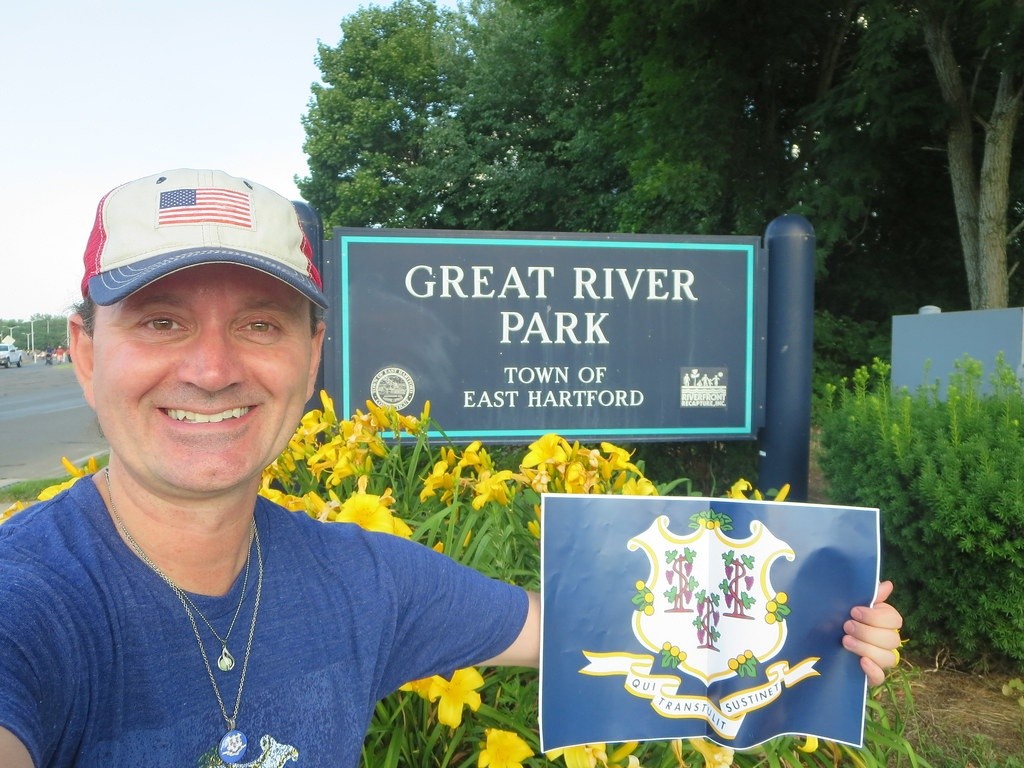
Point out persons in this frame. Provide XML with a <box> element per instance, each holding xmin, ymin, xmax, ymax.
<box><xmin>0</xmin><ymin>166</ymin><xmax>902</xmax><ymax>768</ymax></box>
<box><xmin>26</xmin><ymin>344</ymin><xmax>72</xmax><ymax>366</ymax></box>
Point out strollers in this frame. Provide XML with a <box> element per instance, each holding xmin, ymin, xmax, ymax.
<box><xmin>46</xmin><ymin>354</ymin><xmax>53</xmax><ymax>365</ymax></box>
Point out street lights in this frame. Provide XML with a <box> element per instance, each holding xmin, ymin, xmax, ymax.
<box><xmin>21</xmin><ymin>332</ymin><xmax>35</xmax><ymax>349</ymax></box>
<box><xmin>2</xmin><ymin>325</ymin><xmax>20</xmax><ymax>338</ymax></box>
<box><xmin>22</xmin><ymin>319</ymin><xmax>43</xmax><ymax>352</ymax></box>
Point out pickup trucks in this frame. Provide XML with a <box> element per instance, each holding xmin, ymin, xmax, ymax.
<box><xmin>0</xmin><ymin>344</ymin><xmax>22</xmax><ymax>368</ymax></box>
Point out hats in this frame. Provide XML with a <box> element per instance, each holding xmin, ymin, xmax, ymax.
<box><xmin>80</xmin><ymin>167</ymin><xmax>329</xmax><ymax>309</ymax></box>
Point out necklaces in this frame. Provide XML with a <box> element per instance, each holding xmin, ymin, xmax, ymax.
<box><xmin>103</xmin><ymin>461</ymin><xmax>263</xmax><ymax>762</ymax></box>
